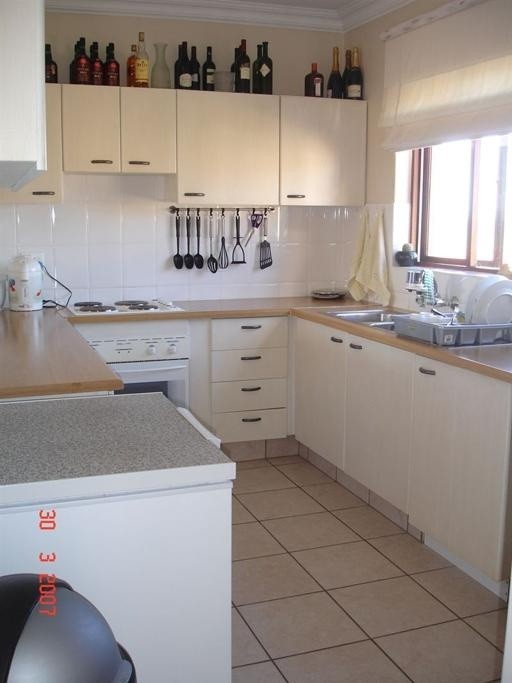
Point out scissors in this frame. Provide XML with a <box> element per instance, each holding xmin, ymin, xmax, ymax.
<box><xmin>245</xmin><ymin>214</ymin><xmax>263</xmax><ymax>248</ymax></box>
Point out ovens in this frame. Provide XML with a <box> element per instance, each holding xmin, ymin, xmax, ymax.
<box><xmin>105</xmin><ymin>359</ymin><xmax>188</xmax><ymax>407</ymax></box>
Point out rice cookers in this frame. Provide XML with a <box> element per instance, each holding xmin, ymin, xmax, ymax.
<box><xmin>6</xmin><ymin>255</ymin><xmax>46</xmax><ymax>311</ymax></box>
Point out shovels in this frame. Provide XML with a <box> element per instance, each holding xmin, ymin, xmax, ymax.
<box><xmin>260</xmin><ymin>211</ymin><xmax>272</xmax><ymax>269</ymax></box>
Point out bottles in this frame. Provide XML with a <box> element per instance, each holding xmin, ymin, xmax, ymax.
<box><xmin>305</xmin><ymin>62</ymin><xmax>324</xmax><ymax>96</ymax></box>
<box><xmin>327</xmin><ymin>46</ymin><xmax>363</xmax><ymax>98</ymax></box>
<box><xmin>70</xmin><ymin>30</ymin><xmax>273</xmax><ymax>94</ymax></box>
<box><xmin>46</xmin><ymin>43</ymin><xmax>58</xmax><ymax>82</ymax></box>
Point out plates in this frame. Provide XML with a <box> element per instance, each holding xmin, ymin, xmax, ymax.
<box><xmin>310</xmin><ymin>288</ymin><xmax>344</xmax><ymax>300</ymax></box>
<box><xmin>465</xmin><ymin>274</ymin><xmax>511</xmax><ymax>324</ymax></box>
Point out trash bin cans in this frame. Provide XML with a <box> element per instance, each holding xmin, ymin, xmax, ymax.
<box><xmin>0</xmin><ymin>575</ymin><xmax>137</xmax><ymax>683</ymax></box>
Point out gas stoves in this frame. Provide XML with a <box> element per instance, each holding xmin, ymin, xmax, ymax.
<box><xmin>66</xmin><ymin>297</ymin><xmax>191</xmax><ymax>360</ymax></box>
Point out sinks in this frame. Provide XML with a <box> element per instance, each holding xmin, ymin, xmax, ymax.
<box><xmin>370</xmin><ymin>324</ymin><xmax>395</xmax><ymax>332</ymax></box>
<box><xmin>323</xmin><ymin>309</ymin><xmax>405</xmax><ymax>323</ymax></box>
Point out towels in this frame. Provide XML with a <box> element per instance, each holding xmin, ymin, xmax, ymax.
<box><xmin>355</xmin><ymin>208</ymin><xmax>394</xmax><ymax>309</ymax></box>
<box><xmin>345</xmin><ymin>210</ymin><xmax>372</xmax><ymax>303</ymax></box>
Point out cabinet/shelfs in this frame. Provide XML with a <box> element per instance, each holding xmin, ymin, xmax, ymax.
<box><xmin>210</xmin><ymin>316</ymin><xmax>289</xmax><ymax>444</ymax></box>
<box><xmin>280</xmin><ymin>94</ymin><xmax>369</xmax><ymax>207</ymax></box>
<box><xmin>295</xmin><ymin>315</ymin><xmax>414</xmax><ymax>514</ymax></box>
<box><xmin>62</xmin><ymin>82</ymin><xmax>178</xmax><ymax>176</ymax></box>
<box><xmin>1</xmin><ymin>82</ymin><xmax>63</xmax><ymax>206</ymax></box>
<box><xmin>1</xmin><ymin>0</ymin><xmax>49</xmax><ymax>194</ymax></box>
<box><xmin>406</xmin><ymin>352</ymin><xmax>510</xmax><ymax>581</ymax></box>
<box><xmin>155</xmin><ymin>88</ymin><xmax>280</xmax><ymax>207</ymax></box>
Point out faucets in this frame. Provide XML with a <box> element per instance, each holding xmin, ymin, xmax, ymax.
<box><xmin>418</xmin><ymin>271</ymin><xmax>440</xmax><ymax>307</ymax></box>
